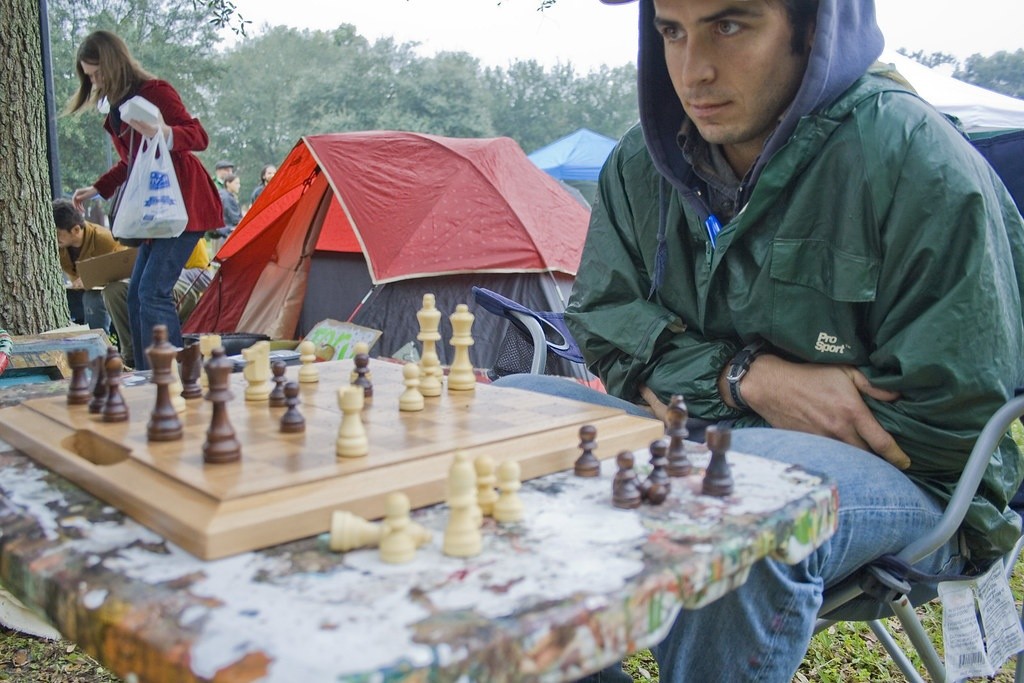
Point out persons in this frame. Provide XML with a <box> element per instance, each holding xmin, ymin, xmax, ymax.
<box><xmin>202</xmin><ymin>162</ymin><xmax>234</xmax><ymax>260</ymax></box>
<box><xmin>58</xmin><ymin>30</ymin><xmax>227</xmax><ymax>370</ymax></box>
<box><xmin>216</xmin><ymin>175</ymin><xmax>243</xmax><ymax>253</ymax></box>
<box><xmin>486</xmin><ymin>0</ymin><xmax>1024</xmax><ymax>683</ymax></box>
<box><xmin>178</xmin><ymin>238</ymin><xmax>214</xmax><ymax>294</ymax></box>
<box><xmin>52</xmin><ymin>198</ymin><xmax>129</xmax><ymax>335</ymax></box>
<box><xmin>250</xmin><ymin>164</ymin><xmax>277</xmax><ymax>206</ymax></box>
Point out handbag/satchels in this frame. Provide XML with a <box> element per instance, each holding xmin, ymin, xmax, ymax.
<box><xmin>111</xmin><ymin>127</ymin><xmax>189</xmax><ymax>239</ymax></box>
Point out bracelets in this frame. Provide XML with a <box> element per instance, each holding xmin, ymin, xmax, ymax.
<box><xmin>725</xmin><ymin>338</ymin><xmax>787</xmax><ymax>415</ymax></box>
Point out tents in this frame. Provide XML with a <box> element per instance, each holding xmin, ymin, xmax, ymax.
<box><xmin>175</xmin><ymin>45</ymin><xmax>1024</xmax><ymax>395</ymax></box>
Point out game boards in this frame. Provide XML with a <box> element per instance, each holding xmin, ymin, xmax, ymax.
<box><xmin>1</xmin><ymin>352</ymin><xmax>669</xmax><ymax>567</ymax></box>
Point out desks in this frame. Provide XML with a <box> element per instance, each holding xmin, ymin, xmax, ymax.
<box><xmin>0</xmin><ymin>355</ymin><xmax>838</xmax><ymax>683</ymax></box>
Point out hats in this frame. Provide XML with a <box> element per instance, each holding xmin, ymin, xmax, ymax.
<box><xmin>216</xmin><ymin>161</ymin><xmax>235</xmax><ymax>169</ymax></box>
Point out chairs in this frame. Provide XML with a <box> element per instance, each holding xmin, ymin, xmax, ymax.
<box><xmin>471</xmin><ymin>124</ymin><xmax>1024</xmax><ymax>683</ymax></box>
